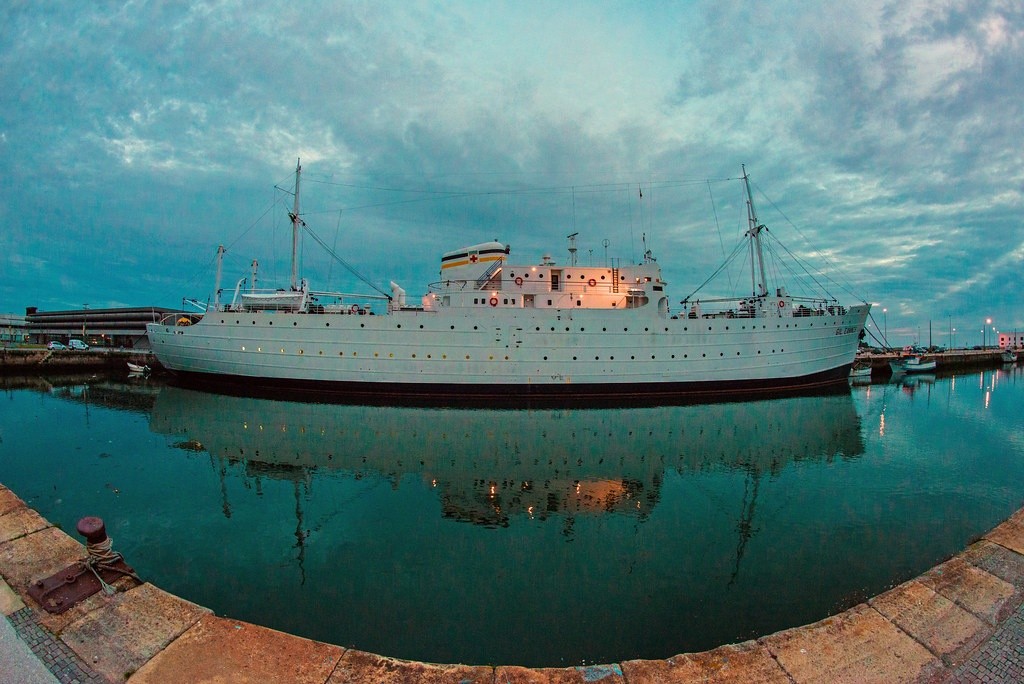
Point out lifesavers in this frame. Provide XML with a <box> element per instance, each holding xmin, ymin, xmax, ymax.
<box><xmin>515</xmin><ymin>276</ymin><xmax>523</xmax><ymax>285</ymax></box>
<box><xmin>351</xmin><ymin>304</ymin><xmax>360</xmax><ymax>314</ymax></box>
<box><xmin>778</xmin><ymin>300</ymin><xmax>784</xmax><ymax>307</ymax></box>
<box><xmin>489</xmin><ymin>298</ymin><xmax>499</xmax><ymax>306</ymax></box>
<box><xmin>588</xmin><ymin>279</ymin><xmax>598</xmax><ymax>287</ymax></box>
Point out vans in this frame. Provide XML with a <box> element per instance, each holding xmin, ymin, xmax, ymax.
<box><xmin>69</xmin><ymin>339</ymin><xmax>89</xmax><ymax>351</ymax></box>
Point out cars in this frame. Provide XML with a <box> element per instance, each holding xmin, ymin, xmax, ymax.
<box><xmin>47</xmin><ymin>341</ymin><xmax>67</xmax><ymax>351</ymax></box>
<box><xmin>914</xmin><ymin>346</ymin><xmax>931</xmax><ymax>354</ymax></box>
<box><xmin>934</xmin><ymin>348</ymin><xmax>944</xmax><ymax>353</ymax></box>
<box><xmin>871</xmin><ymin>348</ymin><xmax>886</xmax><ymax>355</ymax></box>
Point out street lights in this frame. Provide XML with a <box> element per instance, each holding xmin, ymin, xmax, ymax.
<box><xmin>986</xmin><ymin>318</ymin><xmax>991</xmax><ymax>345</ymax></box>
<box><xmin>883</xmin><ymin>308</ymin><xmax>887</xmax><ymax>347</ymax></box>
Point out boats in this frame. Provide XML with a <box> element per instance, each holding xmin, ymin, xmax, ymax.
<box><xmin>900</xmin><ymin>373</ymin><xmax>937</xmax><ymax>388</ymax></box>
<box><xmin>1000</xmin><ymin>349</ymin><xmax>1017</xmax><ymax>363</ymax></box>
<box><xmin>899</xmin><ymin>358</ymin><xmax>938</xmax><ymax>373</ymax></box>
<box><xmin>848</xmin><ymin>360</ymin><xmax>872</xmax><ymax>376</ymax></box>
<box><xmin>127</xmin><ymin>371</ymin><xmax>151</xmax><ymax>379</ymax></box>
<box><xmin>127</xmin><ymin>362</ymin><xmax>152</xmax><ymax>373</ymax></box>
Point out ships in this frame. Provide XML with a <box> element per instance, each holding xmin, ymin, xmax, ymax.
<box><xmin>145</xmin><ymin>156</ymin><xmax>874</xmax><ymax>400</ymax></box>
<box><xmin>150</xmin><ymin>378</ymin><xmax>866</xmax><ymax>592</ymax></box>
